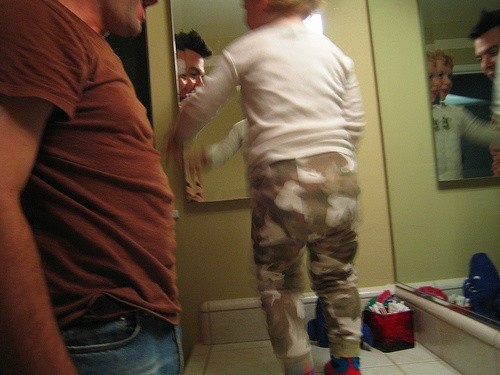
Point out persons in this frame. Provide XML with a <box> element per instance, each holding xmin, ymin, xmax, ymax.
<box><xmin>175</xmin><ymin>29</ymin><xmax>212</xmax><ymax>202</ymax></box>
<box><xmin>200</xmin><ymin>119</ymin><xmax>246</xmax><ymax>171</ymax></box>
<box><xmin>169</xmin><ymin>0</ymin><xmax>367</xmax><ymax>375</ymax></box>
<box><xmin>425</xmin><ymin>49</ymin><xmax>500</xmax><ymax>181</ymax></box>
<box><xmin>467</xmin><ymin>9</ymin><xmax>500</xmax><ymax>177</ymax></box>
<box><xmin>0</xmin><ymin>0</ymin><xmax>184</xmax><ymax>375</ymax></box>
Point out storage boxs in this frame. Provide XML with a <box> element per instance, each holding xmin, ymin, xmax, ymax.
<box><xmin>361</xmin><ymin>307</ymin><xmax>416</xmax><ymax>354</ymax></box>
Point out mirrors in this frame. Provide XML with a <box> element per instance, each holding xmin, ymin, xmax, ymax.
<box><xmin>366</xmin><ymin>0</ymin><xmax>500</xmax><ymax>332</ymax></box>
<box><xmin>168</xmin><ymin>0</ymin><xmax>254</xmax><ymax>206</ymax></box>
<box><xmin>416</xmin><ymin>0</ymin><xmax>500</xmax><ymax>191</ymax></box>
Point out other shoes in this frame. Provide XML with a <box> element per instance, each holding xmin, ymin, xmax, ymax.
<box><xmin>324</xmin><ymin>356</ymin><xmax>361</xmax><ymax>375</ymax></box>
